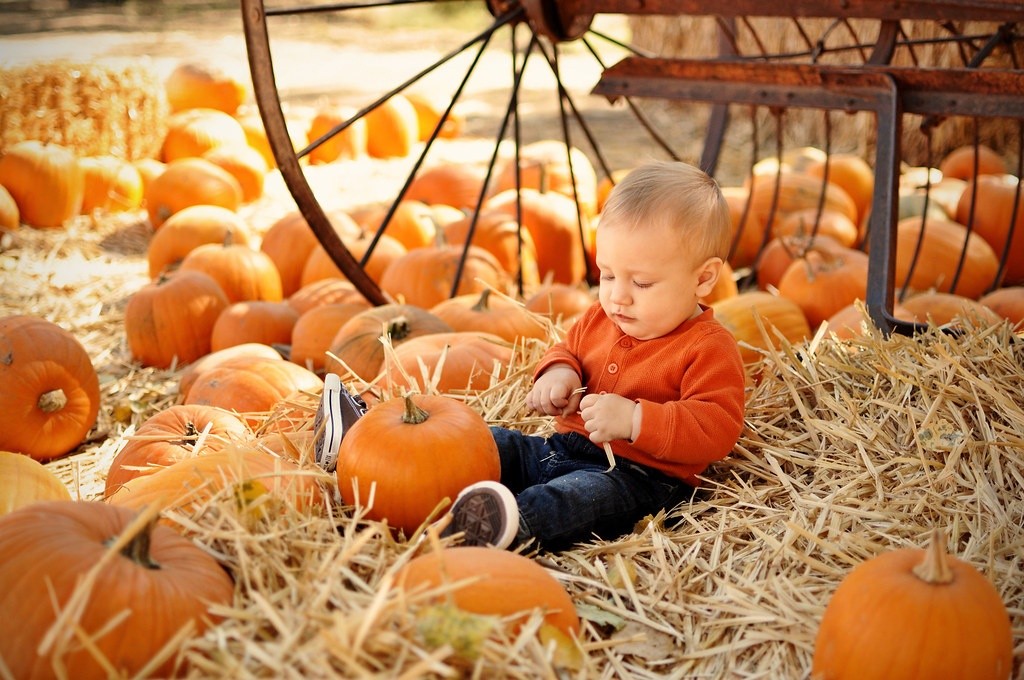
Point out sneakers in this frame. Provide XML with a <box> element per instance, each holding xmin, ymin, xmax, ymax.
<box><xmin>436</xmin><ymin>480</ymin><xmax>519</xmax><ymax>551</ymax></box>
<box><xmin>313</xmin><ymin>372</ymin><xmax>370</xmax><ymax>472</ymax></box>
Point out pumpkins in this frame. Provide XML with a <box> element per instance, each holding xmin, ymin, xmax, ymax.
<box><xmin>0</xmin><ymin>60</ymin><xmax>1023</xmax><ymax>680</ymax></box>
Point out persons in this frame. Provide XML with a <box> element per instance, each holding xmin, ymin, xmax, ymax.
<box><xmin>312</xmin><ymin>162</ymin><xmax>748</xmax><ymax>554</ymax></box>
